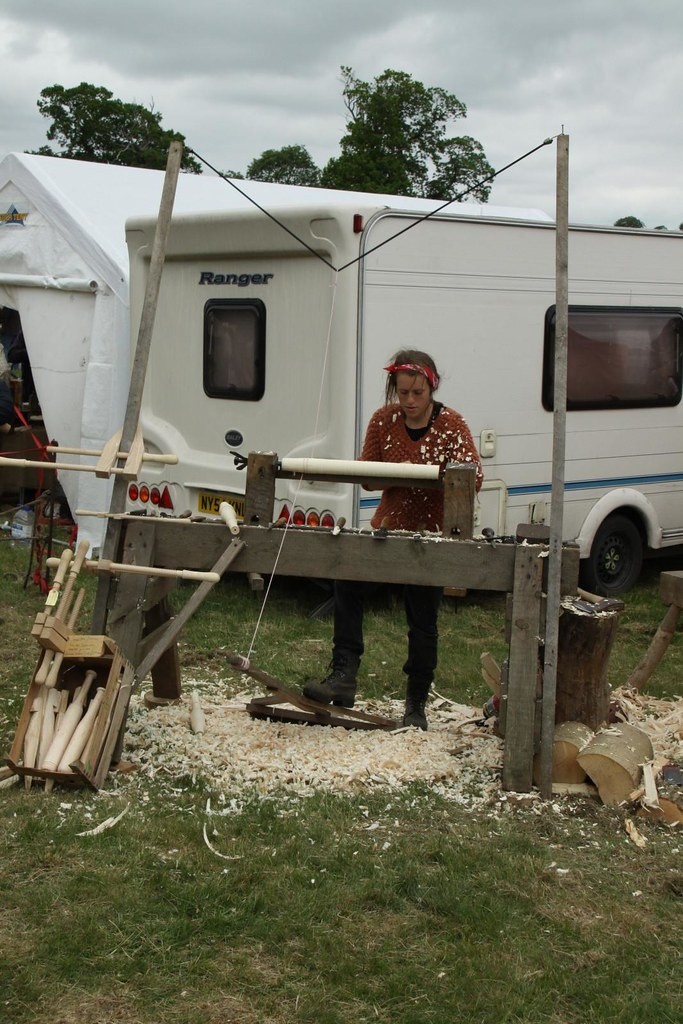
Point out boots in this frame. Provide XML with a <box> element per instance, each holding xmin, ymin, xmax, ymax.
<box><xmin>303</xmin><ymin>651</ymin><xmax>361</xmax><ymax>708</ymax></box>
<box><xmin>403</xmin><ymin>674</ymin><xmax>430</xmax><ymax>731</ymax></box>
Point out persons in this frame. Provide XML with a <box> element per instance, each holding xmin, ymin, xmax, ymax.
<box><xmin>0</xmin><ymin>323</ymin><xmax>33</xmax><ymax>438</ymax></box>
<box><xmin>303</xmin><ymin>349</ymin><xmax>483</xmax><ymax>731</ymax></box>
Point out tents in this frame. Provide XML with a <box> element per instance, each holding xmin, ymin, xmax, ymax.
<box><xmin>0</xmin><ymin>152</ymin><xmax>555</xmax><ymax>517</ymax></box>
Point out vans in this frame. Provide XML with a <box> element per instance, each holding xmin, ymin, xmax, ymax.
<box><xmin>121</xmin><ymin>190</ymin><xmax>683</xmax><ymax>605</ymax></box>
